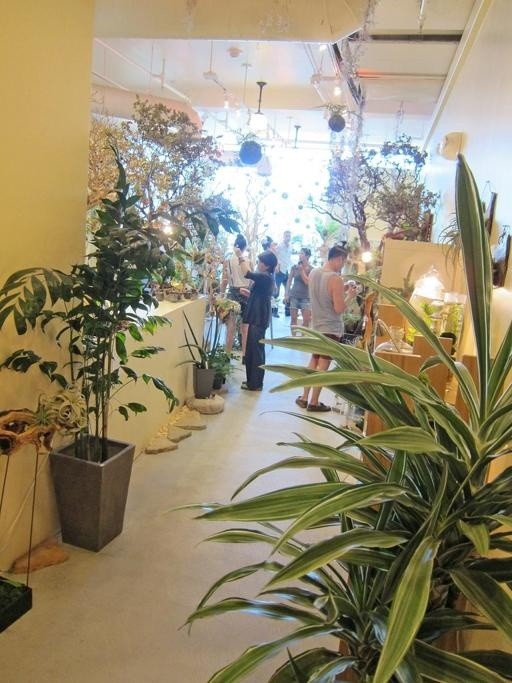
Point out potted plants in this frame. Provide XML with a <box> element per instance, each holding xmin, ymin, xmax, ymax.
<box><xmin>1</xmin><ymin>140</ymin><xmax>177</xmax><ymax>553</ymax></box>
<box><xmin>208</xmin><ymin>344</ymin><xmax>242</xmax><ymax>390</ymax></box>
<box><xmin>172</xmin><ymin>304</ymin><xmax>216</xmax><ymax>399</ymax></box>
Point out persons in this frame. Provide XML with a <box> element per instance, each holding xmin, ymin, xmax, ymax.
<box><xmin>220</xmin><ymin>229</ymin><xmax>359</xmax><ymax>414</ymax></box>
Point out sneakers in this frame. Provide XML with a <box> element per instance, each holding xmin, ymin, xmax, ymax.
<box><xmin>308</xmin><ymin>402</ymin><xmax>331</xmax><ymax>412</ymax></box>
<box><xmin>296</xmin><ymin>395</ymin><xmax>308</xmax><ymax>408</ymax></box>
<box><xmin>241</xmin><ymin>381</ymin><xmax>262</xmax><ymax>391</ymax></box>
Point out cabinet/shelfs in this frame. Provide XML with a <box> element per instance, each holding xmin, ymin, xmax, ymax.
<box><xmin>361</xmin><ymin>300</ymin><xmax>453</xmax><ymax>478</ymax></box>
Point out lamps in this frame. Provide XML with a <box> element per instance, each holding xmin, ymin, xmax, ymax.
<box><xmin>202</xmin><ymin>40</ymin><xmax>302</xmax><ymax>176</ymax></box>
